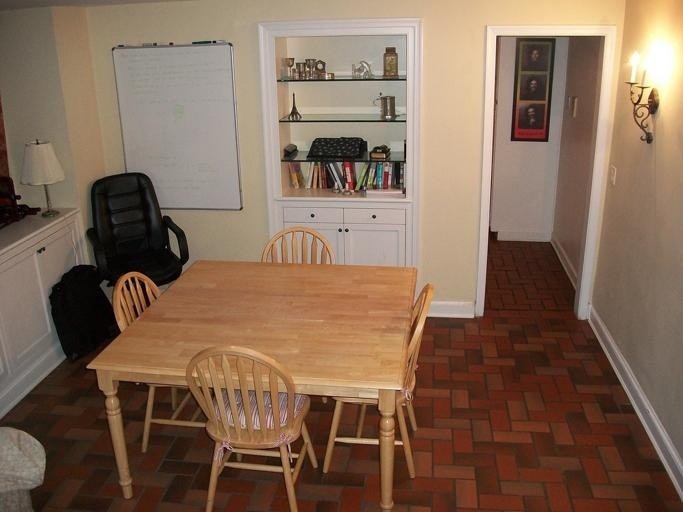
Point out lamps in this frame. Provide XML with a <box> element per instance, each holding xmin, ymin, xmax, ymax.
<box><xmin>20</xmin><ymin>139</ymin><xmax>64</xmax><ymax>218</ymax></box>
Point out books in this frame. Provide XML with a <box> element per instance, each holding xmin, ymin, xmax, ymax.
<box><xmin>286</xmin><ymin>162</ymin><xmax>404</xmax><ymax>197</ymax></box>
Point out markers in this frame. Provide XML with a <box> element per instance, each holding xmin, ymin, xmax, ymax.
<box><xmin>118</xmin><ymin>45</ymin><xmax>124</xmax><ymax>47</ymax></box>
<box><xmin>153</xmin><ymin>43</ymin><xmax>157</xmax><ymax>46</ymax></box>
<box><xmin>143</xmin><ymin>43</ymin><xmax>153</xmax><ymax>46</ymax></box>
<box><xmin>213</xmin><ymin>41</ymin><xmax>216</xmax><ymax>43</ymax></box>
<box><xmin>169</xmin><ymin>42</ymin><xmax>173</xmax><ymax>45</ymax></box>
<box><xmin>192</xmin><ymin>41</ymin><xmax>211</xmax><ymax>44</ymax></box>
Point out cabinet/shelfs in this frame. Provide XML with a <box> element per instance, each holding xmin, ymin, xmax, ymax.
<box><xmin>258</xmin><ymin>19</ymin><xmax>423</xmax><ymax>201</ymax></box>
<box><xmin>0</xmin><ymin>334</ymin><xmax>16</xmax><ymax>419</ymax></box>
<box><xmin>268</xmin><ymin>201</ymin><xmax>419</xmax><ymax>269</ymax></box>
<box><xmin>0</xmin><ymin>208</ymin><xmax>89</xmax><ymax>419</ymax></box>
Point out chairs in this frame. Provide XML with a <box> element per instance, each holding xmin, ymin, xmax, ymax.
<box><xmin>321</xmin><ymin>282</ymin><xmax>435</xmax><ymax>479</ymax></box>
<box><xmin>86</xmin><ymin>172</ymin><xmax>189</xmax><ymax>307</ymax></box>
<box><xmin>262</xmin><ymin>227</ymin><xmax>334</xmax><ymax>265</ymax></box>
<box><xmin>112</xmin><ymin>271</ymin><xmax>208</xmax><ymax>454</ymax></box>
<box><xmin>185</xmin><ymin>344</ymin><xmax>318</xmax><ymax>512</ymax></box>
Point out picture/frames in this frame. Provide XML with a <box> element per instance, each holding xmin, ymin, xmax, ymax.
<box><xmin>511</xmin><ymin>38</ymin><xmax>556</xmax><ymax>142</ymax></box>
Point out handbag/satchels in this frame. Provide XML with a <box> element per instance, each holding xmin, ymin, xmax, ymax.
<box><xmin>49</xmin><ymin>265</ymin><xmax>119</xmax><ymax>362</ymax></box>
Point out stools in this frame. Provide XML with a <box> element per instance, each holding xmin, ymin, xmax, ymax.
<box><xmin>0</xmin><ymin>426</ymin><xmax>46</xmax><ymax>511</ymax></box>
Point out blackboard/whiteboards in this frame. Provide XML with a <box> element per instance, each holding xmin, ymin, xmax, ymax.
<box><xmin>112</xmin><ymin>42</ymin><xmax>243</xmax><ymax>211</ymax></box>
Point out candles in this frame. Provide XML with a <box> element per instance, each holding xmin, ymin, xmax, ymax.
<box><xmin>630</xmin><ymin>51</ymin><xmax>639</xmax><ymax>82</ymax></box>
<box><xmin>641</xmin><ymin>70</ymin><xmax>646</xmax><ymax>85</ymax></box>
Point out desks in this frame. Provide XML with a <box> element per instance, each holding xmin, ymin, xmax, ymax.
<box><xmin>85</xmin><ymin>259</ymin><xmax>418</xmax><ymax>512</ymax></box>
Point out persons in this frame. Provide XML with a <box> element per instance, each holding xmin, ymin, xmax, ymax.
<box><xmin>524</xmin><ymin>105</ymin><xmax>541</xmax><ymax>129</ymax></box>
<box><xmin>526</xmin><ymin>77</ymin><xmax>540</xmax><ymax>97</ymax></box>
<box><xmin>528</xmin><ymin>48</ymin><xmax>542</xmax><ymax>65</ymax></box>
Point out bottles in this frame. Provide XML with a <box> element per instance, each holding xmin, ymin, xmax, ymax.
<box><xmin>382</xmin><ymin>46</ymin><xmax>398</xmax><ymax>77</ymax></box>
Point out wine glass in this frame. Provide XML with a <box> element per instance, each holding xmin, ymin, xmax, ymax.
<box><xmin>284</xmin><ymin>57</ymin><xmax>316</xmax><ymax>79</ymax></box>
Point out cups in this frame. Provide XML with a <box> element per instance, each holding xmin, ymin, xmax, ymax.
<box><xmin>373</xmin><ymin>95</ymin><xmax>396</xmax><ymax>116</ymax></box>
